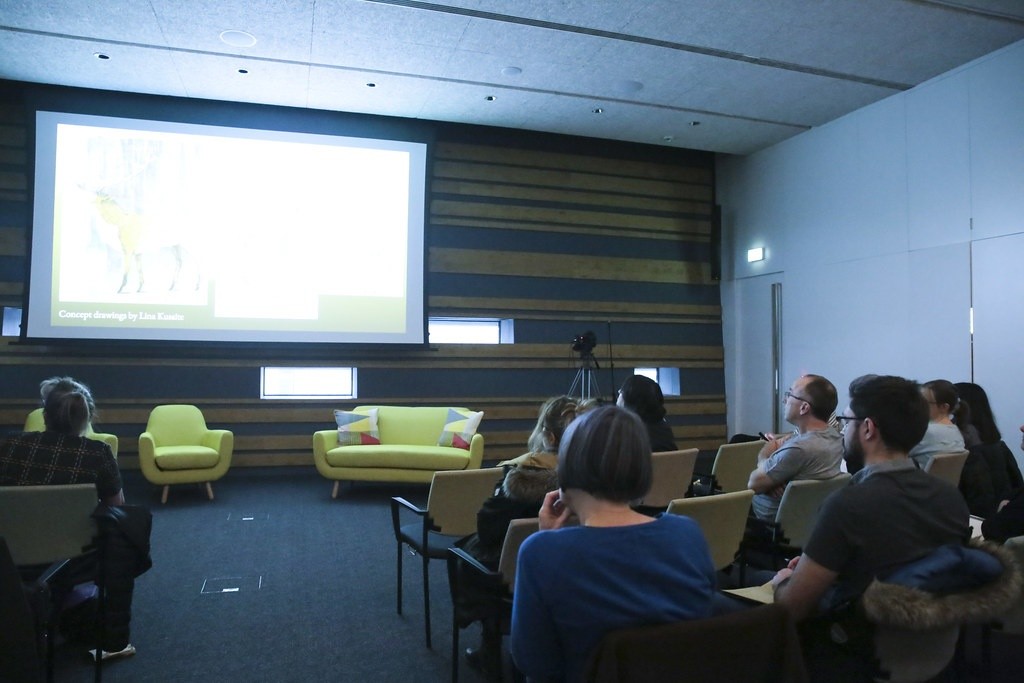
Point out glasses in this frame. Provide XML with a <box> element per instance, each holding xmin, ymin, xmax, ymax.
<box><xmin>785</xmin><ymin>391</ymin><xmax>811</xmax><ymax>406</ymax></box>
<box><xmin>836</xmin><ymin>416</ymin><xmax>878</xmax><ymax>427</ymax></box>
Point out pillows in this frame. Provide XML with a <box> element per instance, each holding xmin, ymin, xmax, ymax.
<box><xmin>438</xmin><ymin>408</ymin><xmax>485</xmax><ymax>450</ymax></box>
<box><xmin>333</xmin><ymin>408</ymin><xmax>380</xmax><ymax>445</ymax></box>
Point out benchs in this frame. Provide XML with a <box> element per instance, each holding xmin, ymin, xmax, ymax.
<box><xmin>313</xmin><ymin>405</ymin><xmax>484</xmax><ymax>498</ymax></box>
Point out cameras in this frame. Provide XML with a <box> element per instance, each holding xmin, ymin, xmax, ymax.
<box><xmin>571</xmin><ymin>332</ymin><xmax>597</xmax><ymax>353</ymax></box>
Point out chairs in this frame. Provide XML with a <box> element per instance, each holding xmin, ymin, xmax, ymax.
<box><xmin>0</xmin><ymin>483</ymin><xmax>151</xmax><ymax>683</ymax></box>
<box><xmin>389</xmin><ymin>431</ymin><xmax>1024</xmax><ymax>683</ymax></box>
<box><xmin>24</xmin><ymin>408</ymin><xmax>118</xmax><ymax>460</ymax></box>
<box><xmin>138</xmin><ymin>404</ymin><xmax>234</xmax><ymax>504</ymax></box>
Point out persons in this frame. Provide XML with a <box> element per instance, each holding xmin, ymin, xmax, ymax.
<box><xmin>717</xmin><ymin>374</ymin><xmax>844</xmax><ymax>588</ymax></box>
<box><xmin>616</xmin><ymin>376</ymin><xmax>679</xmax><ymax>516</ymax></box>
<box><xmin>510</xmin><ymin>405</ymin><xmax>716</xmax><ymax>683</ymax></box>
<box><xmin>447</xmin><ymin>396</ymin><xmax>595</xmax><ymax>681</ymax></box>
<box><xmin>0</xmin><ymin>379</ymin><xmax>134</xmax><ymax>606</ymax></box>
<box><xmin>770</xmin><ymin>375</ymin><xmax>970</xmax><ymax>682</ymax></box>
<box><xmin>911</xmin><ymin>379</ymin><xmax>1024</xmax><ymax>542</ymax></box>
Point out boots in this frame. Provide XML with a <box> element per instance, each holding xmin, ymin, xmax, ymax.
<box><xmin>465</xmin><ymin>636</ymin><xmax>503</xmax><ymax>675</ymax></box>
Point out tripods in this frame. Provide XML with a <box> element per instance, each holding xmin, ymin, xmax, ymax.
<box><xmin>567</xmin><ymin>351</ymin><xmax>601</xmax><ymax>401</ymax></box>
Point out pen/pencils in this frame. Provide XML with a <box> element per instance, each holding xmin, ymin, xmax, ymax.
<box><xmin>759</xmin><ymin>432</ymin><xmax>769</xmax><ymax>441</ymax></box>
<box><xmin>785</xmin><ymin>558</ymin><xmax>790</xmax><ymax>562</ymax></box>
